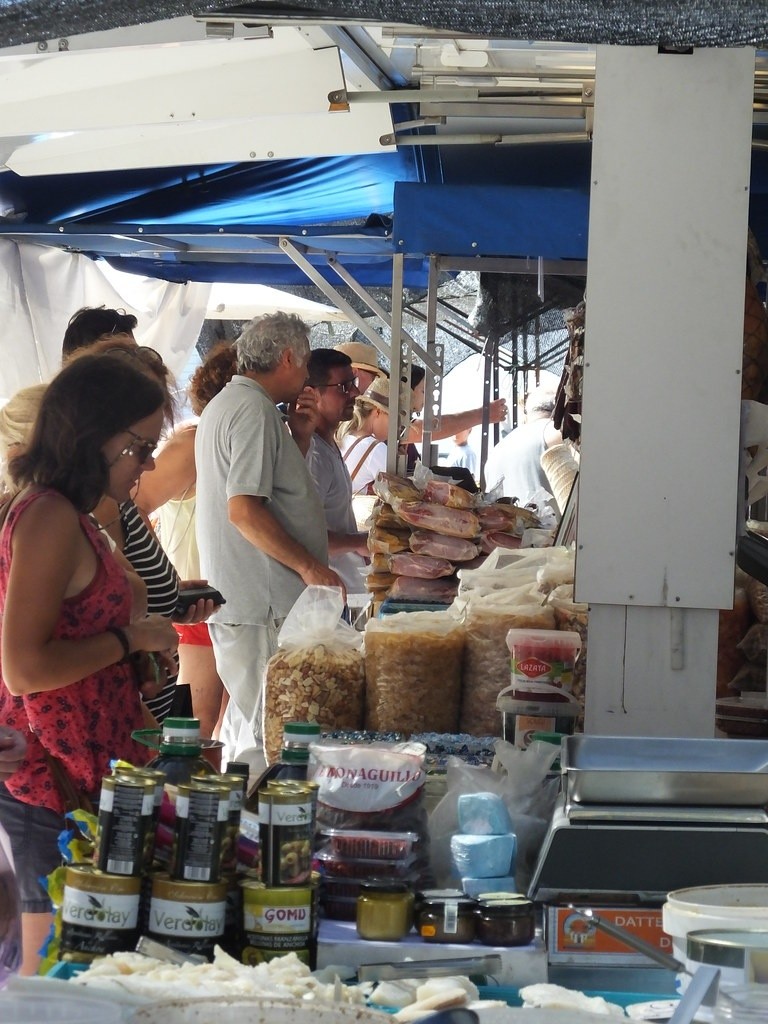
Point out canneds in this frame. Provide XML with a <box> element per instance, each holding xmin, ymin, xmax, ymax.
<box><xmin>412</xmin><ymin>889</ymin><xmax>535</xmax><ymax>948</ymax></box>
<box><xmin>56</xmin><ymin>765</ymin><xmax>319</xmax><ymax>975</ymax></box>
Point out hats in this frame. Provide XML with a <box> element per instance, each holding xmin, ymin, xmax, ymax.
<box><xmin>355</xmin><ymin>377</ymin><xmax>413</xmax><ymax>424</ymax></box>
<box><xmin>334</xmin><ymin>342</ymin><xmax>387</xmax><ymax>379</ymax></box>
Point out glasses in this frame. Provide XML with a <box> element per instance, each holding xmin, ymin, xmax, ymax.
<box><xmin>312</xmin><ymin>376</ymin><xmax>359</xmax><ymax>394</ymax></box>
<box><xmin>120</xmin><ymin>428</ymin><xmax>157</xmax><ymax>465</ymax></box>
<box><xmin>103</xmin><ymin>346</ymin><xmax>162</xmax><ymax>374</ymax></box>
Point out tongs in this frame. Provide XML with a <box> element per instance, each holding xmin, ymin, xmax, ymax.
<box><xmin>356</xmin><ymin>954</ymin><xmax>503</xmax><ymax>982</ymax></box>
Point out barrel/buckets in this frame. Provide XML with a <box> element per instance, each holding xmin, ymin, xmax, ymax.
<box><xmin>1</xmin><ymin>978</ymin><xmax>659</xmax><ymax>1024</ymax></box>
<box><xmin>496</xmin><ymin>683</ymin><xmax>583</xmax><ymax>775</ymax></box>
<box><xmin>662</xmin><ymin>883</ymin><xmax>768</xmax><ymax>995</ymax></box>
<box><xmin>506</xmin><ymin>629</ymin><xmax>581</xmax><ymax>702</ymax></box>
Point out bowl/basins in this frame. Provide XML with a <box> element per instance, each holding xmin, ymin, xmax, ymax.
<box><xmin>314</xmin><ymin>829</ymin><xmax>422</xmax><ymax>920</ymax></box>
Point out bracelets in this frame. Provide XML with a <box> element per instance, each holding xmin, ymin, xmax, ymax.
<box><xmin>106</xmin><ymin>626</ymin><xmax>130</xmax><ymax>662</ymax></box>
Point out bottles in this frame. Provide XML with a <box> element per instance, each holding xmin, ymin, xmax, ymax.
<box><xmin>235</xmin><ymin>721</ymin><xmax>323</xmax><ymax>873</ymax></box>
<box><xmin>131</xmin><ymin>716</ymin><xmax>219</xmax><ymax>833</ymax></box>
<box><xmin>226</xmin><ymin>760</ymin><xmax>249</xmax><ymax>805</ymax></box>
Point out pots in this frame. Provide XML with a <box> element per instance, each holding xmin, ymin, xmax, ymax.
<box><xmin>737</xmin><ymin>519</ymin><xmax>768</xmax><ymax>587</ymax></box>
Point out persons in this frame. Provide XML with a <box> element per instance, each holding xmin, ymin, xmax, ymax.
<box><xmin>484</xmin><ymin>383</ymin><xmax>569</xmax><ymax>529</ymax></box>
<box><xmin>130</xmin><ymin>310</ymin><xmax>511</xmax><ymax>742</ymax></box>
<box><xmin>0</xmin><ymin>303</ymin><xmax>227</xmax><ymax>979</ymax></box>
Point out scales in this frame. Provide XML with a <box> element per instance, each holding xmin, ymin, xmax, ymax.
<box><xmin>528</xmin><ymin>731</ymin><xmax>768</xmax><ymax>905</ymax></box>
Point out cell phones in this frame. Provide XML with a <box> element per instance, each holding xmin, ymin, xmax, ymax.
<box><xmin>178</xmin><ymin>584</ymin><xmax>225</xmax><ymax>609</ymax></box>
